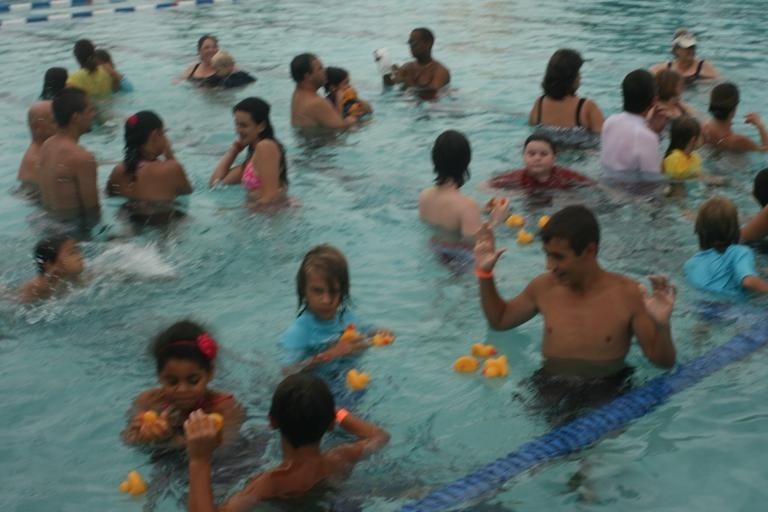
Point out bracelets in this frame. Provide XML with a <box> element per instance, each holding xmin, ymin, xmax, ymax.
<box><xmin>335</xmin><ymin>407</ymin><xmax>352</xmax><ymax>426</ymax></box>
<box><xmin>474</xmin><ymin>266</ymin><xmax>494</xmax><ymax>279</ymax></box>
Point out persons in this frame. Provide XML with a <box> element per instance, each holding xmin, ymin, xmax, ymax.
<box><xmin>739</xmin><ymin>168</ymin><xmax>768</xmax><ymax>240</ymax></box>
<box><xmin>180</xmin><ymin>35</ymin><xmax>222</xmax><ymax>88</ymax></box>
<box><xmin>118</xmin><ymin>319</ymin><xmax>248</xmax><ymax>466</ymax></box>
<box><xmin>17</xmin><ymin>67</ymin><xmax>69</xmax><ymax>183</ymax></box>
<box><xmin>279</xmin><ymin>243</ymin><xmax>390</xmax><ymax>416</ymax></box>
<box><xmin>107</xmin><ymin>110</ymin><xmax>188</xmax><ymax>200</ymax></box>
<box><xmin>681</xmin><ymin>194</ymin><xmax>767</xmax><ymax>295</ymax></box>
<box><xmin>288</xmin><ymin>53</ymin><xmax>372</xmax><ymax>154</ymax></box>
<box><xmin>662</xmin><ymin>115</ymin><xmax>703</xmax><ymax>177</ymax></box>
<box><xmin>526</xmin><ymin>47</ymin><xmax>604</xmax><ymax>152</ymax></box>
<box><xmin>212</xmin><ymin>50</ymin><xmax>257</xmax><ymax>90</ymax></box>
<box><xmin>474</xmin><ymin>206</ymin><xmax>677</xmax><ymax>422</ymax></box>
<box><xmin>184</xmin><ymin>373</ymin><xmax>388</xmax><ymax>512</ymax></box>
<box><xmin>382</xmin><ymin>28</ymin><xmax>450</xmax><ymax>95</ymax></box>
<box><xmin>419</xmin><ymin>129</ymin><xmax>511</xmax><ymax>271</ymax></box>
<box><xmin>648</xmin><ymin>32</ymin><xmax>722</xmax><ymax>83</ymax></box>
<box><xmin>647</xmin><ymin>69</ymin><xmax>696</xmax><ymax>133</ymax></box>
<box><xmin>210</xmin><ymin>96</ymin><xmax>301</xmax><ymax>221</ymax></box>
<box><xmin>701</xmin><ymin>80</ymin><xmax>767</xmax><ymax>151</ymax></box>
<box><xmin>37</xmin><ymin>87</ymin><xmax>100</xmax><ymax>230</ymax></box>
<box><xmin>2</xmin><ymin>235</ymin><xmax>87</xmax><ymax>311</ymax></box>
<box><xmin>65</xmin><ymin>39</ymin><xmax>134</xmax><ymax>99</ymax></box>
<box><xmin>602</xmin><ymin>70</ymin><xmax>663</xmax><ymax>174</ymax></box>
<box><xmin>475</xmin><ymin>134</ymin><xmax>596</xmax><ymax>217</ymax></box>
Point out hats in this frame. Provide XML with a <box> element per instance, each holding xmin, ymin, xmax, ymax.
<box><xmin>672</xmin><ymin>28</ymin><xmax>696</xmax><ymax>49</ymax></box>
